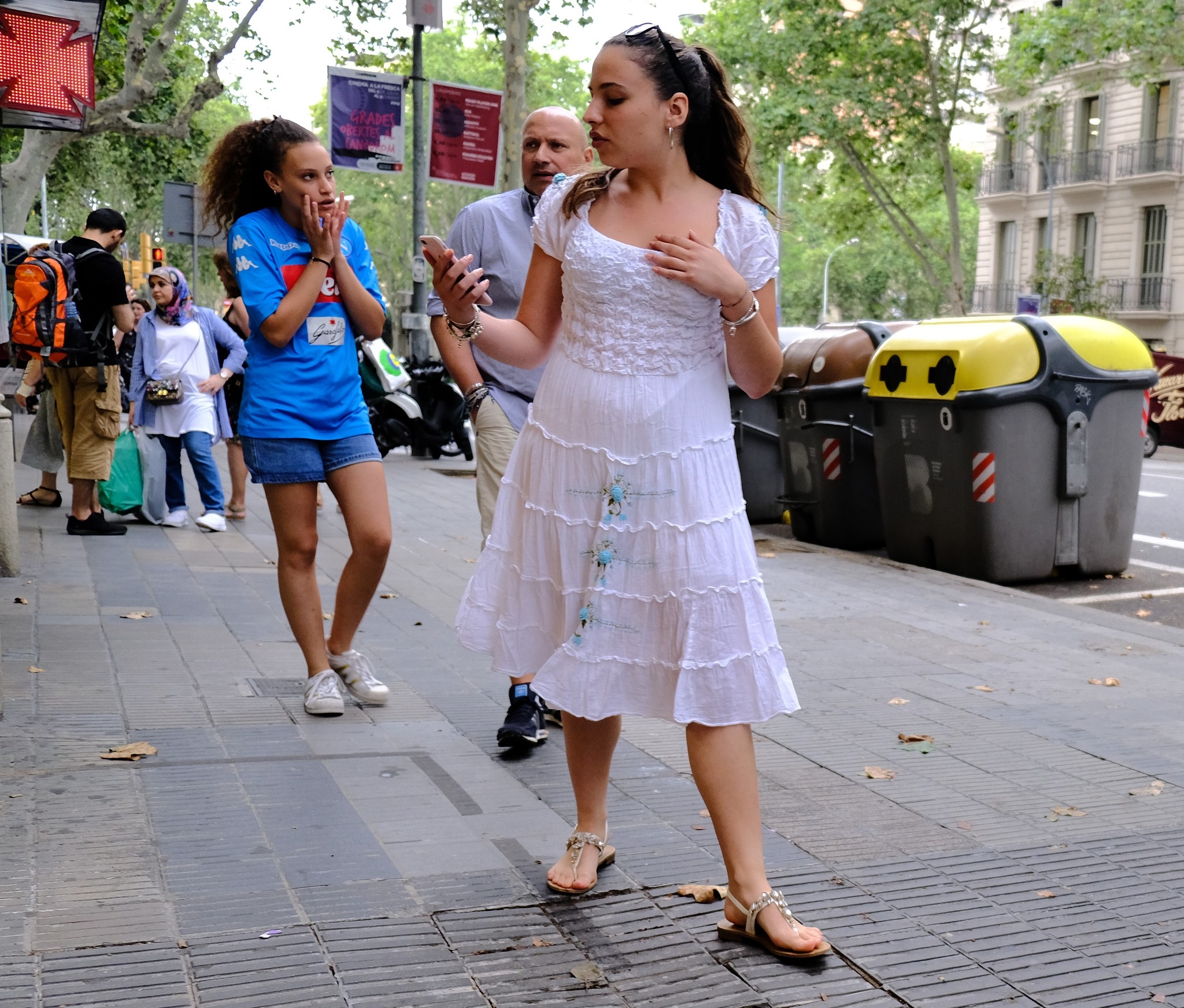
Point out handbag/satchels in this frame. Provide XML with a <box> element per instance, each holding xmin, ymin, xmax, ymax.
<box><xmin>147</xmin><ymin>376</ymin><xmax>184</xmax><ymax>405</ymax></box>
<box><xmin>96</xmin><ymin>424</ymin><xmax>170</xmax><ymax>526</ymax></box>
<box><xmin>361</xmin><ymin>336</ymin><xmax>411</xmax><ymax>393</ymax></box>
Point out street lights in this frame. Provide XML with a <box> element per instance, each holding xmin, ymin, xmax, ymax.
<box><xmin>823</xmin><ymin>237</ymin><xmax>860</xmax><ymax>321</ymax></box>
<box><xmin>986</xmin><ymin>126</ymin><xmax>1056</xmax><ymax>314</ymax></box>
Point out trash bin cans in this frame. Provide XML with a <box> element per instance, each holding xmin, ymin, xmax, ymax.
<box><xmin>767</xmin><ymin>322</ymin><xmax>912</xmax><ymax>547</ymax></box>
<box><xmin>728</xmin><ymin>327</ymin><xmax>809</xmax><ymax>523</ymax></box>
<box><xmin>862</xmin><ymin>311</ymin><xmax>1159</xmax><ymax>589</ymax></box>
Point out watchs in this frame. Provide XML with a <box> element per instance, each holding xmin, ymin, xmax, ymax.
<box><xmin>219</xmin><ymin>369</ymin><xmax>229</xmax><ymax>381</ymax></box>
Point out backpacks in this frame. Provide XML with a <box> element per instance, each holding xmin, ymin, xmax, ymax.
<box><xmin>8</xmin><ymin>241</ymin><xmax>113</xmax><ymax>393</ymax></box>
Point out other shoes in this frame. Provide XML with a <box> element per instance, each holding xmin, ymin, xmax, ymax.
<box><xmin>161</xmin><ymin>509</ymin><xmax>188</xmax><ymax>528</ymax></box>
<box><xmin>195</xmin><ymin>513</ymin><xmax>226</xmax><ymax>532</ymax></box>
<box><xmin>65</xmin><ymin>509</ymin><xmax>128</xmax><ymax>536</ymax></box>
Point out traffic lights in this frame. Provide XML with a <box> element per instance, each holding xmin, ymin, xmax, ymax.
<box><xmin>151</xmin><ymin>247</ymin><xmax>166</xmax><ymax>272</ymax></box>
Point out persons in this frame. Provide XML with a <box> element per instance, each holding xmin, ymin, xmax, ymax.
<box><xmin>200</xmin><ymin>114</ymin><xmax>393</xmax><ymax>715</ymax></box>
<box><xmin>14</xmin><ymin>209</ymin><xmax>342</xmax><ymax>536</ymax></box>
<box><xmin>419</xmin><ymin>22</ymin><xmax>829</xmax><ymax>962</ymax></box>
<box><xmin>427</xmin><ymin>105</ymin><xmax>562</xmax><ymax>748</ymax></box>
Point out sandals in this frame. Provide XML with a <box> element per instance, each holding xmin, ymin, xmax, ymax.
<box><xmin>16</xmin><ymin>486</ymin><xmax>63</xmax><ymax>508</ymax></box>
<box><xmin>200</xmin><ymin>503</ymin><xmax>246</xmax><ymax>521</ymax></box>
<box><xmin>716</xmin><ymin>888</ymin><xmax>832</xmax><ymax>957</ymax></box>
<box><xmin>546</xmin><ymin>820</ymin><xmax>616</xmax><ymax>894</ymax></box>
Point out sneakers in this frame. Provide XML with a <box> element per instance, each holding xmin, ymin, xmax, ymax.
<box><xmin>497</xmin><ymin>681</ymin><xmax>549</xmax><ymax>747</ymax></box>
<box><xmin>304</xmin><ymin>668</ymin><xmax>352</xmax><ymax>714</ymax></box>
<box><xmin>323</xmin><ymin>634</ymin><xmax>390</xmax><ymax>704</ymax></box>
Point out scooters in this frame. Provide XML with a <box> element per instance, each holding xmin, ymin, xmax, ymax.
<box><xmin>355</xmin><ymin>351</ymin><xmax>476</xmax><ymax>462</ymax></box>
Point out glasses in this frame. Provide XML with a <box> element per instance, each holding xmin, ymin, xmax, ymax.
<box><xmin>611</xmin><ymin>23</ymin><xmax>696</xmax><ymax>93</ymax></box>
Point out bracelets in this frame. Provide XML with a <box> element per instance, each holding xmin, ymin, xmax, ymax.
<box><xmin>442</xmin><ymin>303</ymin><xmax>484</xmax><ymax>348</ymax></box>
<box><xmin>310</xmin><ymin>256</ymin><xmax>331</xmax><ymax>268</ymax></box>
<box><xmin>718</xmin><ymin>281</ymin><xmax>759</xmax><ymax>337</ymax></box>
<box><xmin>460</xmin><ymin>383</ymin><xmax>494</xmax><ymax>421</ymax></box>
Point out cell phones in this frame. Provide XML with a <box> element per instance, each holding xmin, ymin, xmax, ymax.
<box><xmin>418</xmin><ymin>235</ymin><xmax>494</xmax><ymax>306</ymax></box>
